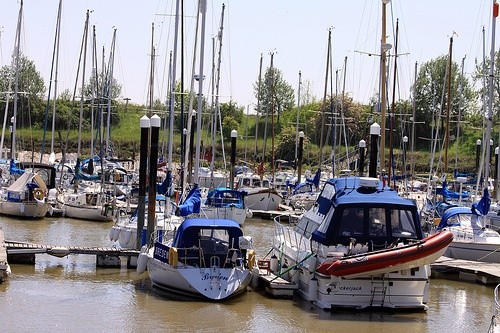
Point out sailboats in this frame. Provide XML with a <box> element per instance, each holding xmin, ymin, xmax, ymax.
<box><xmin>0</xmin><ymin>0</ymin><xmax>500</xmax><ymax>315</ymax></box>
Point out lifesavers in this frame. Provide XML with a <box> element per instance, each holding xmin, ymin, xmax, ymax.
<box><xmin>31</xmin><ymin>187</ymin><xmax>44</xmax><ymax>199</ymax></box>
<box><xmin>168</xmin><ymin>247</ymin><xmax>178</xmax><ymax>267</ymax></box>
<box><xmin>248</xmin><ymin>249</ymin><xmax>256</xmax><ymax>271</ymax></box>
